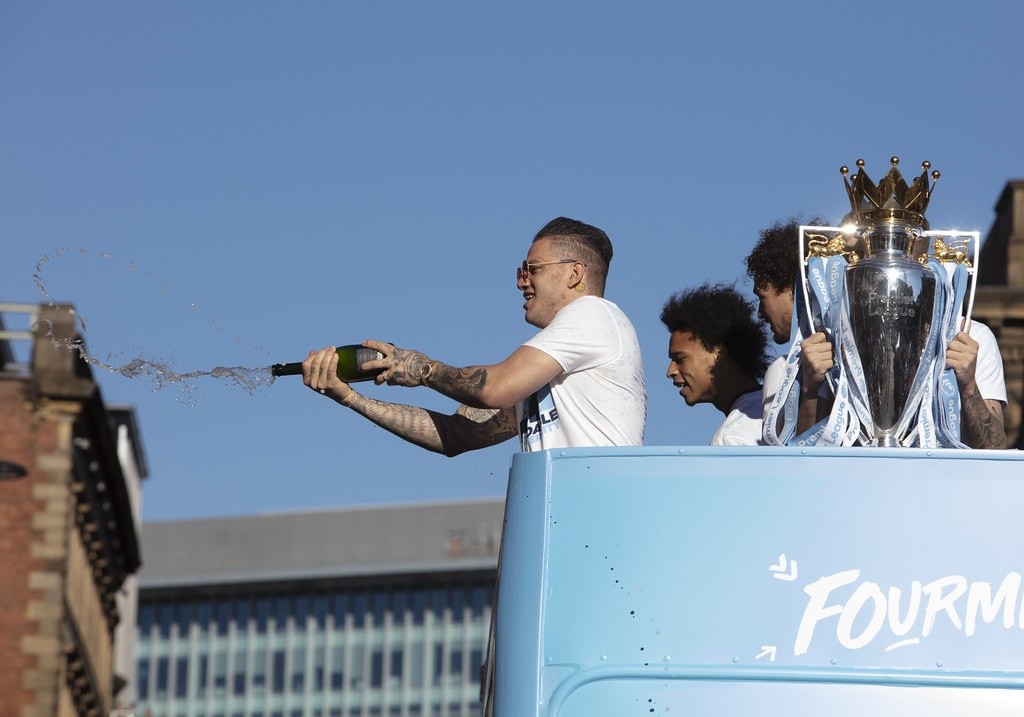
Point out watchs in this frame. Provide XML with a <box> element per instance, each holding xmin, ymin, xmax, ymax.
<box><xmin>420</xmin><ymin>360</ymin><xmax>438</xmax><ymax>388</ymax></box>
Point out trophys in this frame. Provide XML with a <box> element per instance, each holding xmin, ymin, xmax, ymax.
<box><xmin>798</xmin><ymin>155</ymin><xmax>981</xmax><ymax>445</ymax></box>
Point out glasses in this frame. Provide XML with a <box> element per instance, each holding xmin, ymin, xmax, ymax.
<box><xmin>517</xmin><ymin>260</ymin><xmax>587</xmax><ymax>281</ymax></box>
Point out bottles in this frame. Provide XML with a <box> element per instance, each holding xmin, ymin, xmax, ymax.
<box><xmin>272</xmin><ymin>343</ymin><xmax>395</xmax><ymax>382</ymax></box>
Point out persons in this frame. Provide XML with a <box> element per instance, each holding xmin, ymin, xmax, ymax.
<box><xmin>301</xmin><ymin>214</ymin><xmax>648</xmax><ymax>459</ymax></box>
<box><xmin>746</xmin><ymin>216</ymin><xmax>836</xmax><ymax>346</ymax></box>
<box><xmin>760</xmin><ymin>211</ymin><xmax>1008</xmax><ymax>449</ymax></box>
<box><xmin>657</xmin><ymin>279</ymin><xmax>771</xmax><ymax>447</ymax></box>
